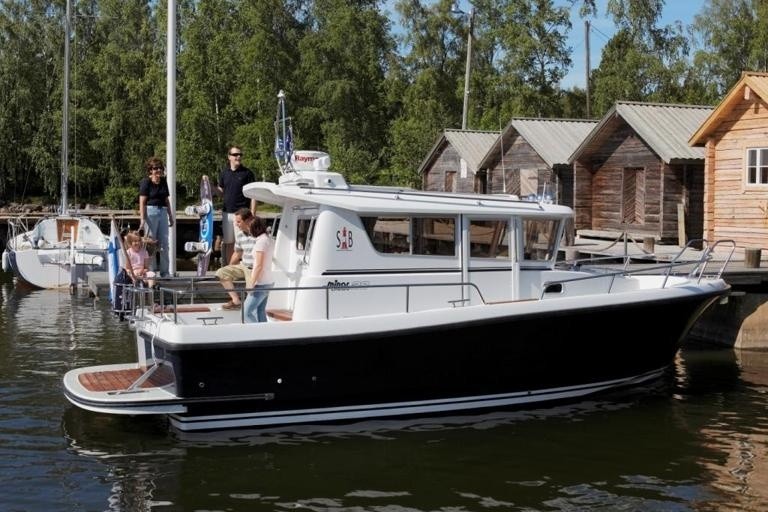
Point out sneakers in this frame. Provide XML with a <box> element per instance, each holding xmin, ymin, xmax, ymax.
<box><xmin>160</xmin><ymin>274</ymin><xmax>174</xmax><ymax>280</ymax></box>
<box><xmin>223</xmin><ymin>301</ymin><xmax>242</xmax><ymax>311</ymax></box>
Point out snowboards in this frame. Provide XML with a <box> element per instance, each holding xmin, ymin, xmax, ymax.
<box><xmin>185</xmin><ymin>174</ymin><xmax>214</xmax><ymax>277</ymax></box>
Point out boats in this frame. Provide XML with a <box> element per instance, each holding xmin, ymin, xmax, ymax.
<box><xmin>85</xmin><ymin>262</ymin><xmax>254</xmax><ymax>303</ymax></box>
<box><xmin>2</xmin><ymin>216</ymin><xmax>124</xmax><ymax>289</ymax></box>
<box><xmin>61</xmin><ymin>147</ymin><xmax>739</xmax><ymax>433</ymax></box>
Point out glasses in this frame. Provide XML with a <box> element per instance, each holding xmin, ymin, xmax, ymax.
<box><xmin>153</xmin><ymin>167</ymin><xmax>163</xmax><ymax>170</ymax></box>
<box><xmin>229</xmin><ymin>153</ymin><xmax>242</xmax><ymax>156</ymax></box>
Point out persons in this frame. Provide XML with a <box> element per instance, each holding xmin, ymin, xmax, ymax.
<box><xmin>200</xmin><ymin>145</ymin><xmax>257</xmax><ymax>266</ymax></box>
<box><xmin>125</xmin><ymin>231</ymin><xmax>157</xmax><ymax>289</ymax></box>
<box><xmin>138</xmin><ymin>157</ymin><xmax>175</xmax><ymax>284</ymax></box>
<box><xmin>238</xmin><ymin>216</ymin><xmax>276</xmax><ymax>322</ymax></box>
<box><xmin>213</xmin><ymin>207</ymin><xmax>255</xmax><ymax>312</ymax></box>
